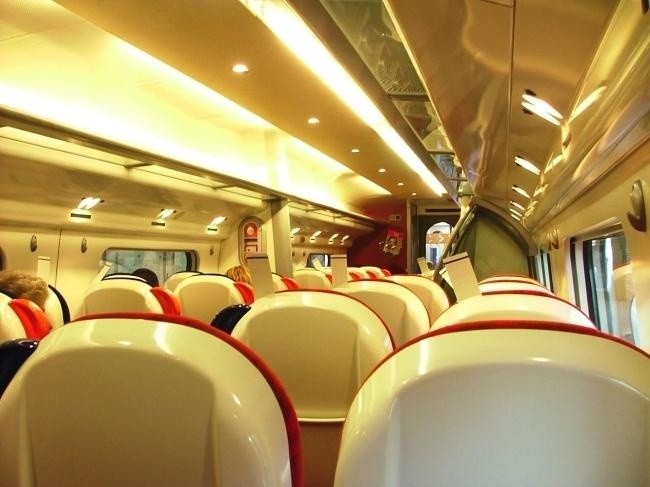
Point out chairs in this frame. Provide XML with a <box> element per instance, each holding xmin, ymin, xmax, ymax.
<box><xmin>430</xmin><ymin>296</ymin><xmax>474</xmax><ymax>332</ymax></box>
<box><xmin>164</xmin><ymin>271</ymin><xmax>255</xmax><ymax>325</ymax></box>
<box><xmin>0</xmin><ymin>312</ymin><xmax>305</xmax><ymax>487</ymax></box>
<box><xmin>230</xmin><ymin>291</ymin><xmax>389</xmax><ymax>487</ymax></box>
<box><xmin>77</xmin><ymin>273</ymin><xmax>182</xmax><ymax>317</ymax></box>
<box><xmin>334</xmin><ymin>322</ymin><xmax>475</xmax><ymax>487</ymax></box>
<box><xmin>383</xmin><ymin>274</ymin><xmax>449</xmax><ymax>326</ymax></box>
<box><xmin>270</xmin><ymin>270</ymin><xmax>307</xmax><ymax>297</ymax></box>
<box><xmin>293</xmin><ymin>267</ymin><xmax>391</xmax><ymax>291</ymax></box>
<box><xmin>335</xmin><ymin>279</ymin><xmax>430</xmax><ymax>349</ymax></box>
<box><xmin>0</xmin><ymin>292</ymin><xmax>52</xmax><ymax>343</ymax></box>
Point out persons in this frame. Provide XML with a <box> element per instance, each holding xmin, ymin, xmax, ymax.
<box><xmin>226</xmin><ymin>265</ymin><xmax>252</xmax><ymax>285</ymax></box>
<box><xmin>0</xmin><ymin>268</ymin><xmax>48</xmax><ymax>313</ymax></box>
<box><xmin>383</xmin><ymin>236</ymin><xmax>401</xmax><ymax>255</ymax></box>
<box><xmin>132</xmin><ymin>268</ymin><xmax>159</xmax><ymax>288</ymax></box>
